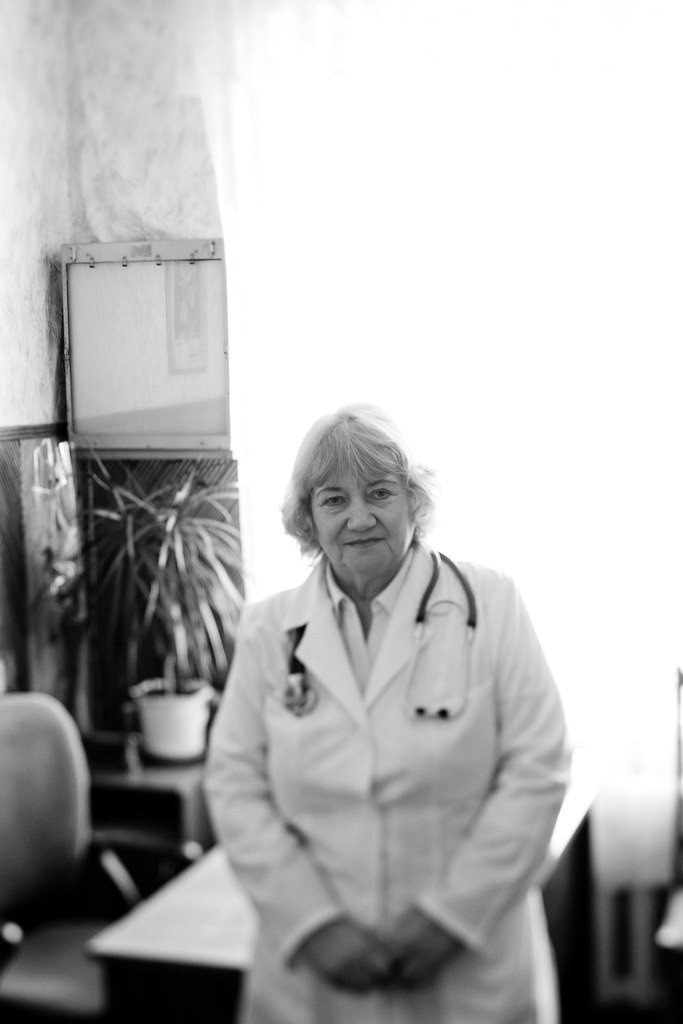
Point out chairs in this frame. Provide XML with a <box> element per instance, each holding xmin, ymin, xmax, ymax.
<box><xmin>0</xmin><ymin>691</ymin><xmax>142</xmax><ymax>1018</ymax></box>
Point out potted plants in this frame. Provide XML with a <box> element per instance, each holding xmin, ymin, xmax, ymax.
<box><xmin>62</xmin><ymin>441</ymin><xmax>242</xmax><ymax>762</ymax></box>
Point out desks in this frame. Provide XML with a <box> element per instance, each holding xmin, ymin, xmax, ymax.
<box><xmin>87</xmin><ymin>795</ymin><xmax>596</xmax><ymax>1024</ymax></box>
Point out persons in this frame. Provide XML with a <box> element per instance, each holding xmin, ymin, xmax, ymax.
<box><xmin>204</xmin><ymin>401</ymin><xmax>572</xmax><ymax>1024</ymax></box>
